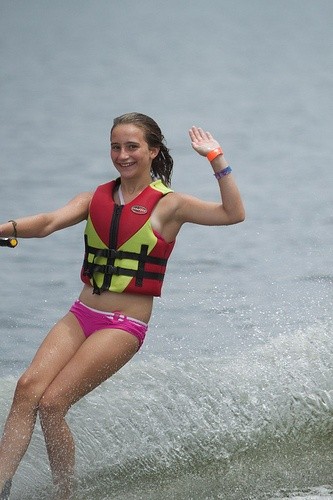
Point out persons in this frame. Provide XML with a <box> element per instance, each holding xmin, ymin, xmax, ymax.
<box><xmin>0</xmin><ymin>111</ymin><xmax>246</xmax><ymax>500</ymax></box>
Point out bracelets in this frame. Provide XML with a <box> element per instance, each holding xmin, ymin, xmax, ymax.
<box><xmin>213</xmin><ymin>165</ymin><xmax>232</xmax><ymax>181</ymax></box>
<box><xmin>8</xmin><ymin>219</ymin><xmax>18</xmax><ymax>238</ymax></box>
<box><xmin>207</xmin><ymin>148</ymin><xmax>222</xmax><ymax>162</ymax></box>
